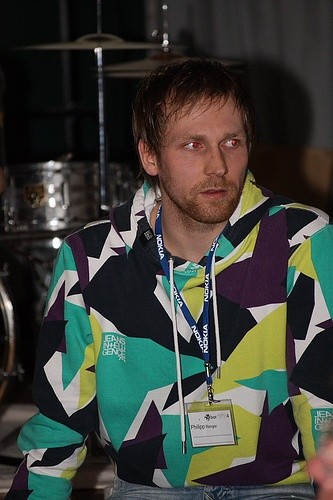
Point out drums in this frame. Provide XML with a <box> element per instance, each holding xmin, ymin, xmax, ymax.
<box><xmin>0</xmin><ymin>283</ymin><xmax>26</xmax><ymax>396</ymax></box>
<box><xmin>97</xmin><ymin>165</ymin><xmax>134</xmax><ymax>210</ymax></box>
<box><xmin>3</xmin><ymin>159</ymin><xmax>102</xmax><ymax>234</ymax></box>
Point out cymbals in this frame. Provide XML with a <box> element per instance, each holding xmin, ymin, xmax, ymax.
<box><xmin>103</xmin><ymin>52</ymin><xmax>241</xmax><ymax>79</ymax></box>
<box><xmin>11</xmin><ymin>33</ymin><xmax>164</xmax><ymax>50</ymax></box>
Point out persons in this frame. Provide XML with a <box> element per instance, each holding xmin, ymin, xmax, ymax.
<box><xmin>1</xmin><ymin>57</ymin><xmax>332</xmax><ymax>500</ymax></box>
